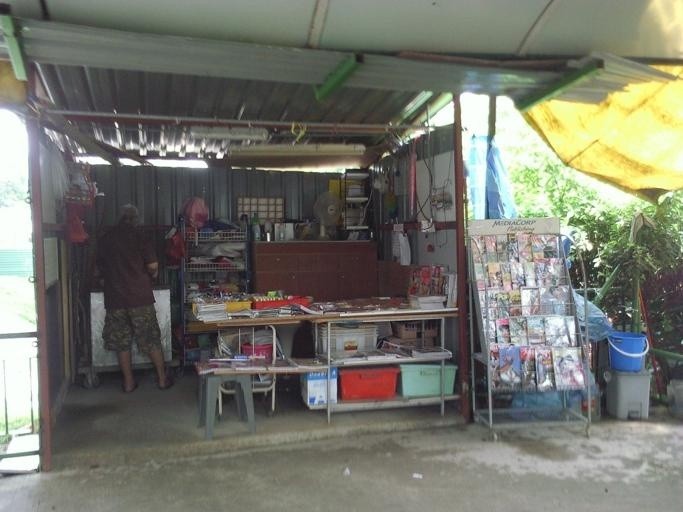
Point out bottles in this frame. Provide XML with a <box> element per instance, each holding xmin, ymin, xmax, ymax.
<box><xmin>278</xmin><ymin>222</ymin><xmax>285</xmax><ymax>241</ymax></box>
<box><xmin>251</xmin><ymin>212</ymin><xmax>260</xmax><ymax>241</ymax></box>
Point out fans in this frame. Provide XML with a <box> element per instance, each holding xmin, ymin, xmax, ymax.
<box><xmin>313</xmin><ymin>193</ymin><xmax>342</xmax><ymax>239</ymax></box>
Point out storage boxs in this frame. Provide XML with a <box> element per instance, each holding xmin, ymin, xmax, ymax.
<box><xmin>299</xmin><ymin>363</ymin><xmax>457</xmax><ymax>405</ymax></box>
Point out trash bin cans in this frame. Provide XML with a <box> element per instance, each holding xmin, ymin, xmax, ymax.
<box><xmin>606</xmin><ymin>372</ymin><xmax>650</xmax><ymax>419</ymax></box>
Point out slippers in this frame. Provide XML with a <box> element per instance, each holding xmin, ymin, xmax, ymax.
<box><xmin>121</xmin><ymin>377</ymin><xmax>174</xmax><ymax>393</ymax></box>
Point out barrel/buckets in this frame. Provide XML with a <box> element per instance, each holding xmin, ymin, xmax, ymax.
<box><xmin>605</xmin><ymin>331</ymin><xmax>650</xmax><ymax>371</ymax></box>
<box><xmin>241</xmin><ymin>344</ymin><xmax>275</xmax><ymax>363</ymax></box>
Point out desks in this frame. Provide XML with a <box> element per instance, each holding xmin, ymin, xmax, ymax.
<box><xmin>198</xmin><ymin>299</ymin><xmax>460</xmax><ymax>419</ymax></box>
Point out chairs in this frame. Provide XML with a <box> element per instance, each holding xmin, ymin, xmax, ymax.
<box><xmin>215</xmin><ymin>325</ymin><xmax>275</xmax><ymax>414</ymax></box>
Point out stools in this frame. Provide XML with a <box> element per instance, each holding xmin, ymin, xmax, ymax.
<box><xmin>198</xmin><ymin>372</ymin><xmax>253</xmax><ymax>433</ymax></box>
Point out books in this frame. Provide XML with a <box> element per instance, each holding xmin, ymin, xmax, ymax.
<box><xmin>193</xmin><ymin>340</ymin><xmax>454</xmax><ymax>376</ymax></box>
<box><xmin>192</xmin><ymin>296</ymin><xmax>323</xmax><ymax>324</ymax></box>
<box><xmin>407</xmin><ymin>263</ymin><xmax>457</xmax><ymax>309</ymax></box>
<box><xmin>469</xmin><ymin>233</ymin><xmax>587</xmax><ymax>391</ymax></box>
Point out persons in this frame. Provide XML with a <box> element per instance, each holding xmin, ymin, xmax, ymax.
<box><xmin>96</xmin><ymin>203</ymin><xmax>175</xmax><ymax>393</ymax></box>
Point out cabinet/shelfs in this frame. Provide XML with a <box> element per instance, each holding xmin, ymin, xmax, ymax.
<box><xmin>178</xmin><ymin>217</ymin><xmax>249</xmax><ymax>370</ymax></box>
<box><xmin>340</xmin><ymin>170</ymin><xmax>371</xmax><ymax>230</ymax></box>
